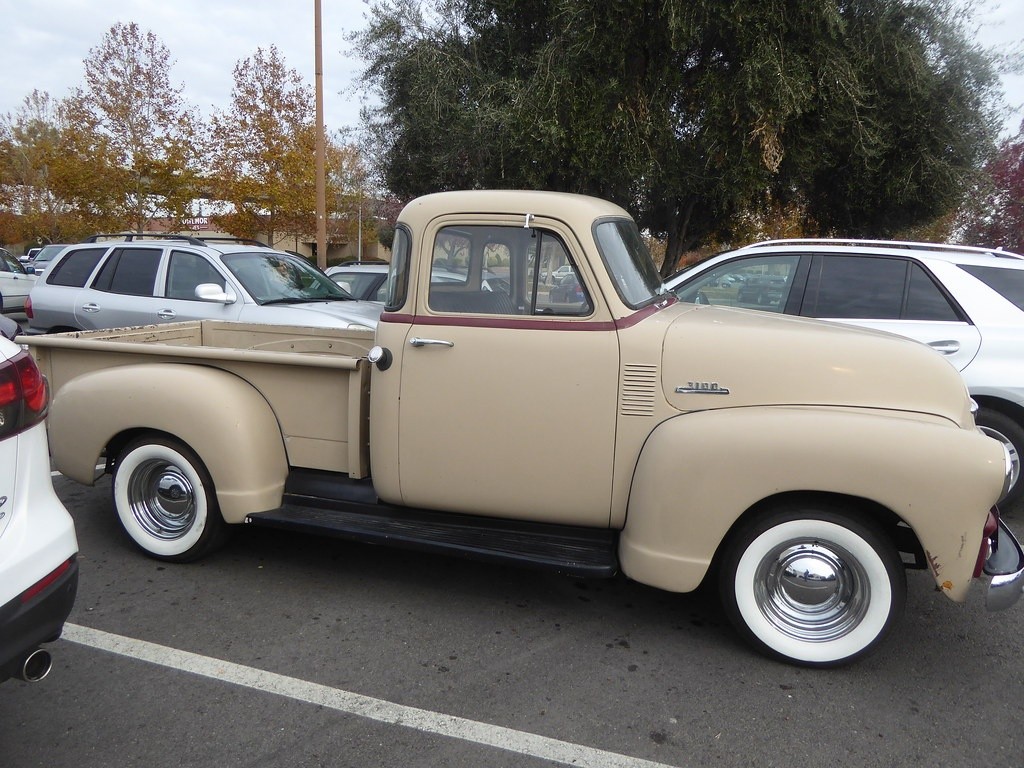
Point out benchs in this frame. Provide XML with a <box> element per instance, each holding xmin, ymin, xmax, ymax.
<box><xmin>430</xmin><ymin>291</ymin><xmax>519</xmax><ymax>313</ymax></box>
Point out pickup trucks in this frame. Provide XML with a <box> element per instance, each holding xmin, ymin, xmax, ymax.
<box><xmin>12</xmin><ymin>184</ymin><xmax>1024</xmax><ymax>672</ymax></box>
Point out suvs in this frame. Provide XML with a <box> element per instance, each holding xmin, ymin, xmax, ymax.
<box><xmin>0</xmin><ymin>230</ymin><xmax>545</xmax><ymax>396</ymax></box>
<box><xmin>655</xmin><ymin>236</ymin><xmax>1024</xmax><ymax>534</ymax></box>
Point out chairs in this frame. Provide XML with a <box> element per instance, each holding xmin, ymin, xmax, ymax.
<box><xmin>174</xmin><ymin>264</ymin><xmax>197</xmax><ymax>301</ymax></box>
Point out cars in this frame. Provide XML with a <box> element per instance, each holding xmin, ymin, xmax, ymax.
<box><xmin>0</xmin><ymin>331</ymin><xmax>81</xmax><ymax>686</ymax></box>
